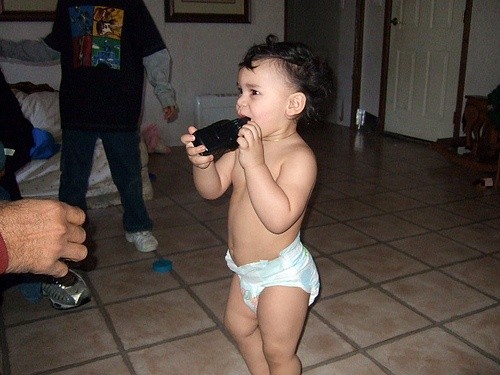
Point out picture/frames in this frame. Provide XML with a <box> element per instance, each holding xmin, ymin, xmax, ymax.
<box><xmin>164</xmin><ymin>0</ymin><xmax>252</xmax><ymax>24</ymax></box>
<box><xmin>0</xmin><ymin>0</ymin><xmax>57</xmax><ymax>22</ymax></box>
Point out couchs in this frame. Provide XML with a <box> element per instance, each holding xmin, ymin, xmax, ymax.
<box><xmin>10</xmin><ymin>87</ymin><xmax>154</xmax><ymax>209</ymax></box>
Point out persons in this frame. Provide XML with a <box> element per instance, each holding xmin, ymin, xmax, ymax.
<box><xmin>0</xmin><ymin>62</ymin><xmax>92</xmax><ymax>310</ymax></box>
<box><xmin>180</xmin><ymin>35</ymin><xmax>330</xmax><ymax>375</ymax></box>
<box><xmin>0</xmin><ymin>197</ymin><xmax>88</xmax><ymax>279</ymax></box>
<box><xmin>41</xmin><ymin>0</ymin><xmax>179</xmax><ymax>252</ymax></box>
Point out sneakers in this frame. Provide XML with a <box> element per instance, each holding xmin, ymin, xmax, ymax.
<box><xmin>41</xmin><ymin>270</ymin><xmax>91</xmax><ymax>310</ymax></box>
<box><xmin>125</xmin><ymin>230</ymin><xmax>159</xmax><ymax>251</ymax></box>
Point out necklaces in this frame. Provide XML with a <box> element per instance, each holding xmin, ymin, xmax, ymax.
<box><xmin>260</xmin><ymin>131</ymin><xmax>296</xmax><ymax>142</ymax></box>
